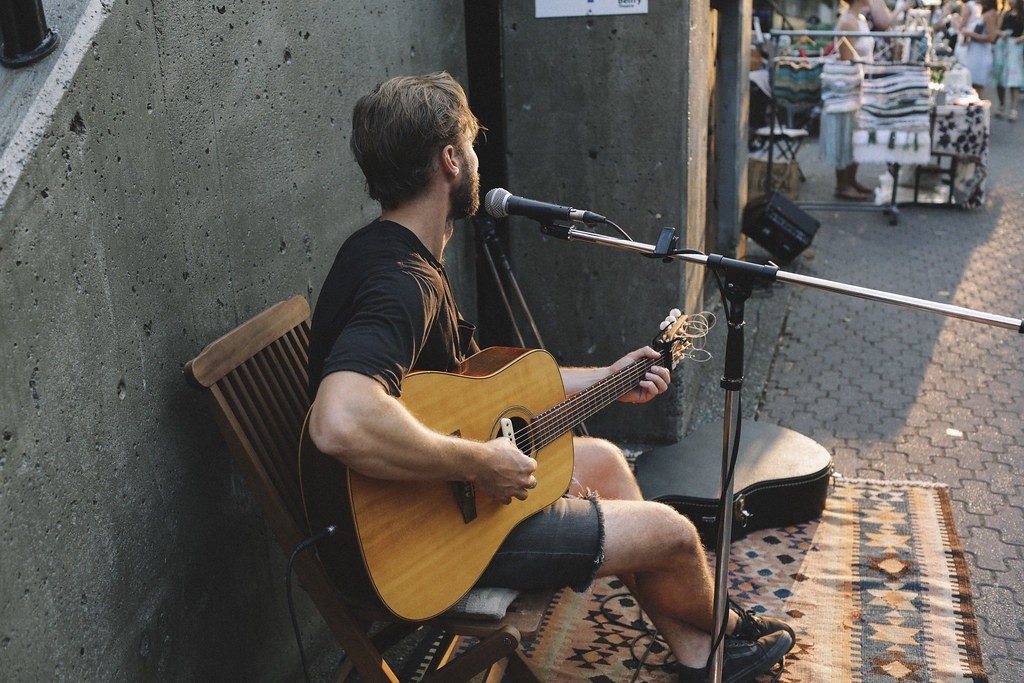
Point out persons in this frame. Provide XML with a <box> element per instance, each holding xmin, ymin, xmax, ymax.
<box><xmin>816</xmin><ymin>0</ymin><xmax>1024</xmax><ymax>200</ymax></box>
<box><xmin>310</xmin><ymin>73</ymin><xmax>798</xmax><ymax>683</ymax></box>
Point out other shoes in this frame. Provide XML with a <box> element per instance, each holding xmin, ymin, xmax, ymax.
<box><xmin>995</xmin><ymin>106</ymin><xmax>1005</xmax><ymax>118</ymax></box>
<box><xmin>1008</xmin><ymin>109</ymin><xmax>1018</xmax><ymax>121</ymax></box>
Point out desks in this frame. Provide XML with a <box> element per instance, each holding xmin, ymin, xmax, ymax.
<box><xmin>888</xmin><ymin>93</ymin><xmax>992</xmax><ymax>209</ymax></box>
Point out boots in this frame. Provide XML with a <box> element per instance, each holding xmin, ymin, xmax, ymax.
<box><xmin>835</xmin><ymin>162</ymin><xmax>876</xmax><ymax>201</ymax></box>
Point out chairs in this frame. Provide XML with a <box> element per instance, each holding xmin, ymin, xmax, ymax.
<box><xmin>183</xmin><ymin>294</ymin><xmax>557</xmax><ymax>683</ymax></box>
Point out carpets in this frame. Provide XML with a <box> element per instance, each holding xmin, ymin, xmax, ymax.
<box><xmin>405</xmin><ymin>451</ymin><xmax>988</xmax><ymax>683</ymax></box>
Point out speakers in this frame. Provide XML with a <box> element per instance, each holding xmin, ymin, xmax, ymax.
<box><xmin>740</xmin><ymin>191</ymin><xmax>821</xmax><ymax>264</ymax></box>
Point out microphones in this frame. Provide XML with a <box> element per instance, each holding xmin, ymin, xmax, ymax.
<box><xmin>485</xmin><ymin>188</ymin><xmax>607</xmax><ymax>224</ymax></box>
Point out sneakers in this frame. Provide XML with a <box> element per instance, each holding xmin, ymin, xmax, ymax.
<box><xmin>679</xmin><ymin>598</ymin><xmax>796</xmax><ymax>683</ymax></box>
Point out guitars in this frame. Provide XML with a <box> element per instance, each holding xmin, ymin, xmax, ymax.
<box><xmin>294</xmin><ymin>306</ymin><xmax>717</xmax><ymax>628</ymax></box>
